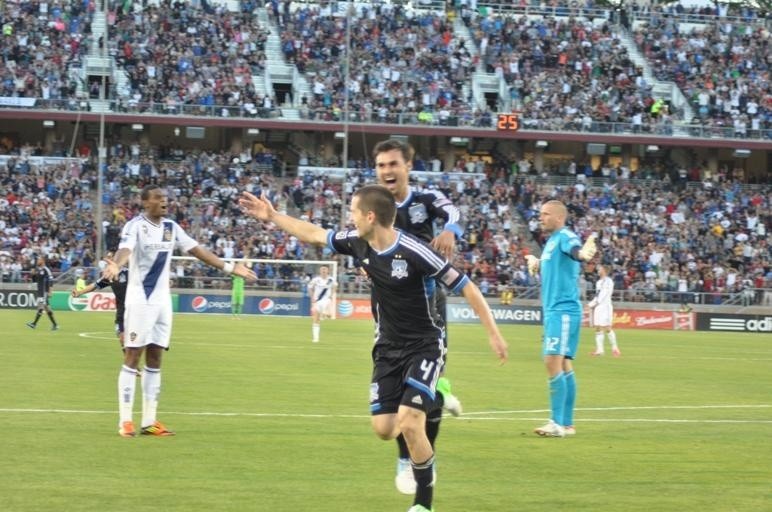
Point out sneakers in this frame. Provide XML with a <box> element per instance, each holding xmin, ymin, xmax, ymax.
<box><xmin>396</xmin><ymin>459</ymin><xmax>417</xmax><ymax>494</ymax></box>
<box><xmin>534</xmin><ymin>419</ymin><xmax>564</xmax><ymax>438</ymax></box>
<box><xmin>51</xmin><ymin>325</ymin><xmax>58</xmax><ymax>330</ymax></box>
<box><xmin>407</xmin><ymin>504</ymin><xmax>434</xmax><ymax>512</ymax></box>
<box><xmin>436</xmin><ymin>377</ymin><xmax>462</xmax><ymax>417</ymax></box>
<box><xmin>26</xmin><ymin>322</ymin><xmax>36</xmax><ymax>329</ymax></box>
<box><xmin>563</xmin><ymin>425</ymin><xmax>575</xmax><ymax>434</ymax></box>
<box><xmin>591</xmin><ymin>349</ymin><xmax>603</xmax><ymax>355</ymax></box>
<box><xmin>612</xmin><ymin>347</ymin><xmax>619</xmax><ymax>357</ymax></box>
<box><xmin>135</xmin><ymin>367</ymin><xmax>143</xmax><ymax>377</ymax></box>
<box><xmin>118</xmin><ymin>422</ymin><xmax>137</xmax><ymax>437</ymax></box>
<box><xmin>142</xmin><ymin>420</ymin><xmax>174</xmax><ymax>436</ymax></box>
<box><xmin>312</xmin><ymin>338</ymin><xmax>319</xmax><ymax>343</ymax></box>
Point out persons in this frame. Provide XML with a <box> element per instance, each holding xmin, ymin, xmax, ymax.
<box><xmin>616</xmin><ymin>1</ymin><xmax>772</xmax><ymax>137</ymax></box>
<box><xmin>26</xmin><ymin>254</ymin><xmax>59</xmax><ymax>330</ymax></box>
<box><xmin>352</xmin><ymin>141</ymin><xmax>467</xmax><ymax>496</ymax></box>
<box><xmin>449</xmin><ymin>0</ymin><xmax>673</xmax><ymax>135</ymax></box>
<box><xmin>102</xmin><ymin>186</ymin><xmax>258</xmax><ymax>437</ymax></box>
<box><xmin>0</xmin><ymin>0</ymin><xmax>263</xmax><ymax>116</ymax></box>
<box><xmin>71</xmin><ymin>258</ymin><xmax>129</xmax><ymax>352</ymax></box>
<box><xmin>588</xmin><ymin>265</ymin><xmax>620</xmax><ymax>357</ymax></box>
<box><xmin>239</xmin><ymin>186</ymin><xmax>509</xmax><ymax>512</ymax></box>
<box><xmin>264</xmin><ymin>0</ymin><xmax>492</xmax><ymax>126</ymax></box>
<box><xmin>515</xmin><ymin>142</ymin><xmax>772</xmax><ymax>303</ymax></box>
<box><xmin>534</xmin><ymin>200</ymin><xmax>597</xmax><ymax>438</ymax></box>
<box><xmin>289</xmin><ymin>139</ymin><xmax>539</xmax><ymax>296</ymax></box>
<box><xmin>307</xmin><ymin>265</ymin><xmax>340</xmax><ymax>343</ymax></box>
<box><xmin>1</xmin><ymin>139</ymin><xmax>307</xmax><ymax>289</ymax></box>
